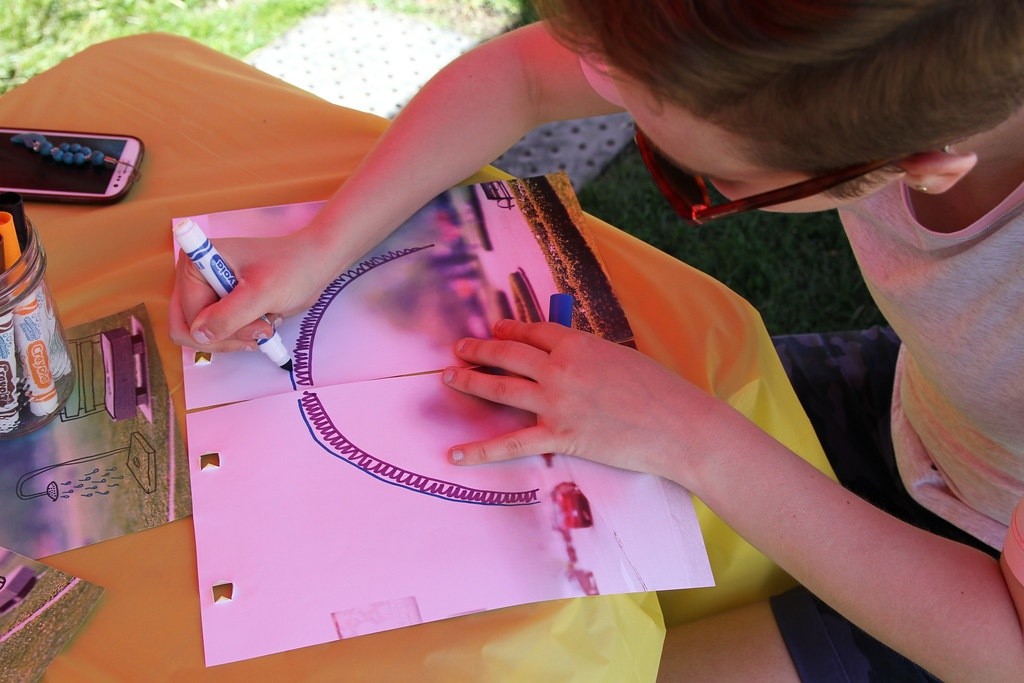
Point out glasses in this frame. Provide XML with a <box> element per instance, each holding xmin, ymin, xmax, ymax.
<box><xmin>634</xmin><ymin>123</ymin><xmax>968</xmax><ymax>230</ymax></box>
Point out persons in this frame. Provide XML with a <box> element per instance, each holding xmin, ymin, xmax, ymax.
<box><xmin>168</xmin><ymin>0</ymin><xmax>1024</xmax><ymax>683</ymax></box>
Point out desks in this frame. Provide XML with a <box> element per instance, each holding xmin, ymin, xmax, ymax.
<box><xmin>1</xmin><ymin>34</ymin><xmax>842</xmax><ymax>682</ymax></box>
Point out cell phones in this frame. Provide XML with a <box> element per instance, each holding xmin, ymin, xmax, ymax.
<box><xmin>0</xmin><ymin>127</ymin><xmax>145</xmax><ymax>206</ymax></box>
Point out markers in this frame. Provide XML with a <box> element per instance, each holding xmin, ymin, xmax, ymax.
<box><xmin>174</xmin><ymin>218</ymin><xmax>295</xmax><ymax>376</ymax></box>
<box><xmin>0</xmin><ymin>190</ymin><xmax>71</xmax><ymax>438</ymax></box>
